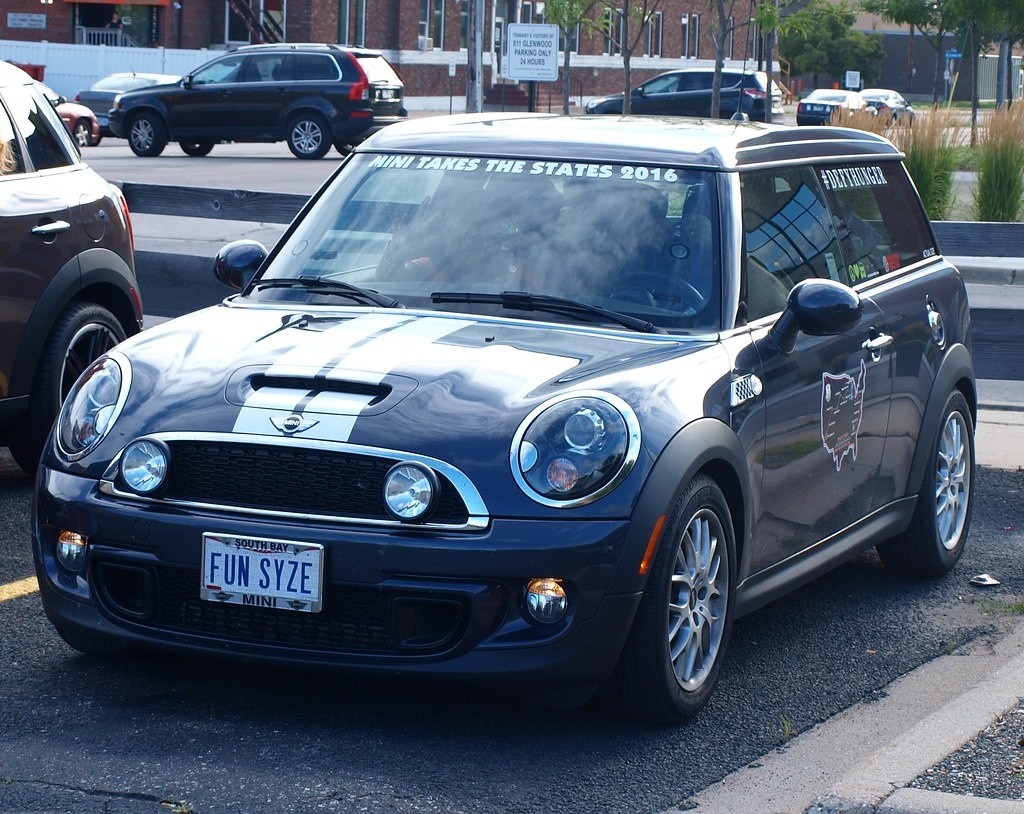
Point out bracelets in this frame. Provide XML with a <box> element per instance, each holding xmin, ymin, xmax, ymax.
<box><xmin>382</xmin><ymin>254</ymin><xmax>404</xmax><ymax>269</ymax></box>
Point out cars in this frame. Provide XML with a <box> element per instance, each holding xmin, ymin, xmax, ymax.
<box><xmin>796</xmin><ymin>88</ymin><xmax>878</xmax><ymax>126</ymax></box>
<box><xmin>34</xmin><ymin>81</ymin><xmax>101</xmax><ymax>146</ymax></box>
<box><xmin>74</xmin><ymin>72</ymin><xmax>182</xmax><ymax>138</ymax></box>
<box><xmin>859</xmin><ymin>88</ymin><xmax>916</xmax><ymax>129</ymax></box>
<box><xmin>31</xmin><ymin>110</ymin><xmax>979</xmax><ymax>728</ymax></box>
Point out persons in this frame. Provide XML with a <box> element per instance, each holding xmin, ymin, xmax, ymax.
<box><xmin>375</xmin><ymin>190</ymin><xmax>523</xmax><ymax>292</ymax></box>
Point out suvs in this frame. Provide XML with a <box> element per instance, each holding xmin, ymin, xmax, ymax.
<box><xmin>0</xmin><ymin>61</ymin><xmax>144</xmax><ymax>479</ymax></box>
<box><xmin>584</xmin><ymin>68</ymin><xmax>785</xmax><ymax>124</ymax></box>
<box><xmin>108</xmin><ymin>41</ymin><xmax>409</xmax><ymax>161</ymax></box>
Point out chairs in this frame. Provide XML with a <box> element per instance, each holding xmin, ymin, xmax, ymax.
<box><xmin>485</xmin><ymin>168</ymin><xmax>886</xmax><ymax>311</ymax></box>
